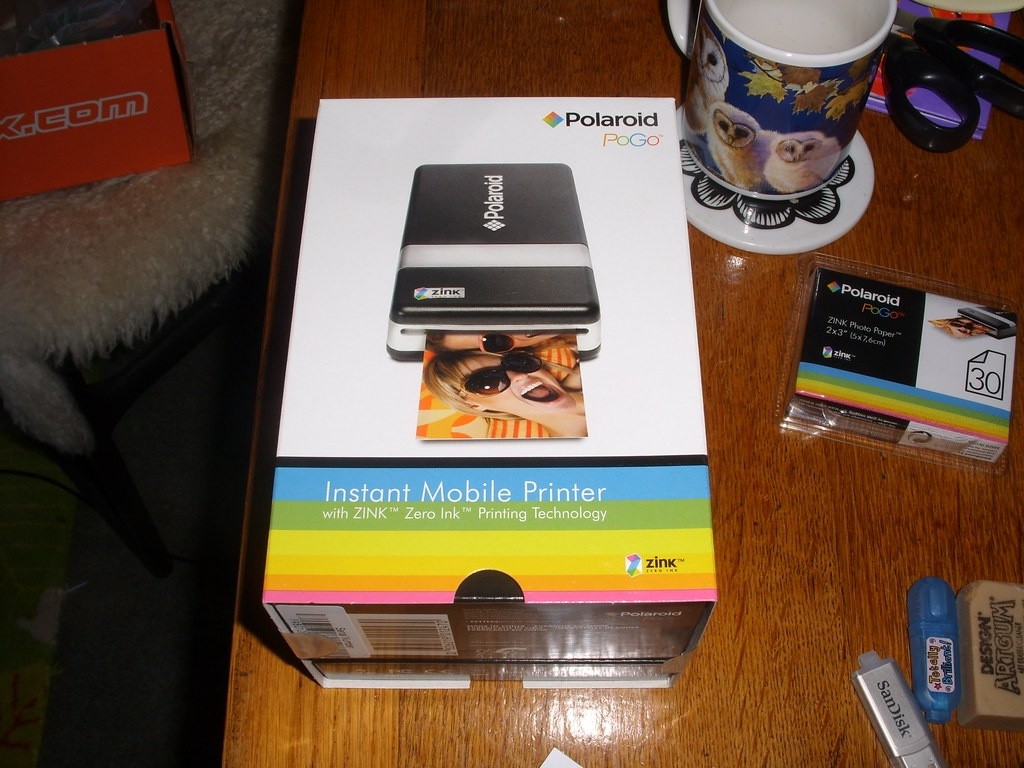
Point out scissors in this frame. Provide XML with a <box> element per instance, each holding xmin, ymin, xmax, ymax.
<box><xmin>881</xmin><ymin>18</ymin><xmax>1024</xmax><ymax>155</ymax></box>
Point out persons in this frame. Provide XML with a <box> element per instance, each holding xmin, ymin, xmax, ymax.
<box><xmin>423</xmin><ymin>330</ymin><xmax>588</xmax><ymax>437</ymax></box>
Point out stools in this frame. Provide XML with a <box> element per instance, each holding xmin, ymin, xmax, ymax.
<box><xmin>0</xmin><ymin>0</ymin><xmax>304</xmax><ymax>573</ymax></box>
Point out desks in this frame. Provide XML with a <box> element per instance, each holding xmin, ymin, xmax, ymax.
<box><xmin>225</xmin><ymin>1</ymin><xmax>1024</xmax><ymax>768</ymax></box>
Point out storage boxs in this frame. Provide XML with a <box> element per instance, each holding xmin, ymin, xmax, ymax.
<box><xmin>263</xmin><ymin>95</ymin><xmax>722</xmax><ymax>688</ymax></box>
<box><xmin>1</xmin><ymin>0</ymin><xmax>199</xmax><ymax>200</ymax></box>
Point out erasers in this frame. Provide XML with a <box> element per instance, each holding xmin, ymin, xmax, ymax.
<box><xmin>958</xmin><ymin>580</ymin><xmax>1024</xmax><ymax>731</ymax></box>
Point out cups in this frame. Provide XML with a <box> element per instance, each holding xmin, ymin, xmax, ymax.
<box><xmin>667</xmin><ymin>0</ymin><xmax>897</xmax><ymax>200</ymax></box>
